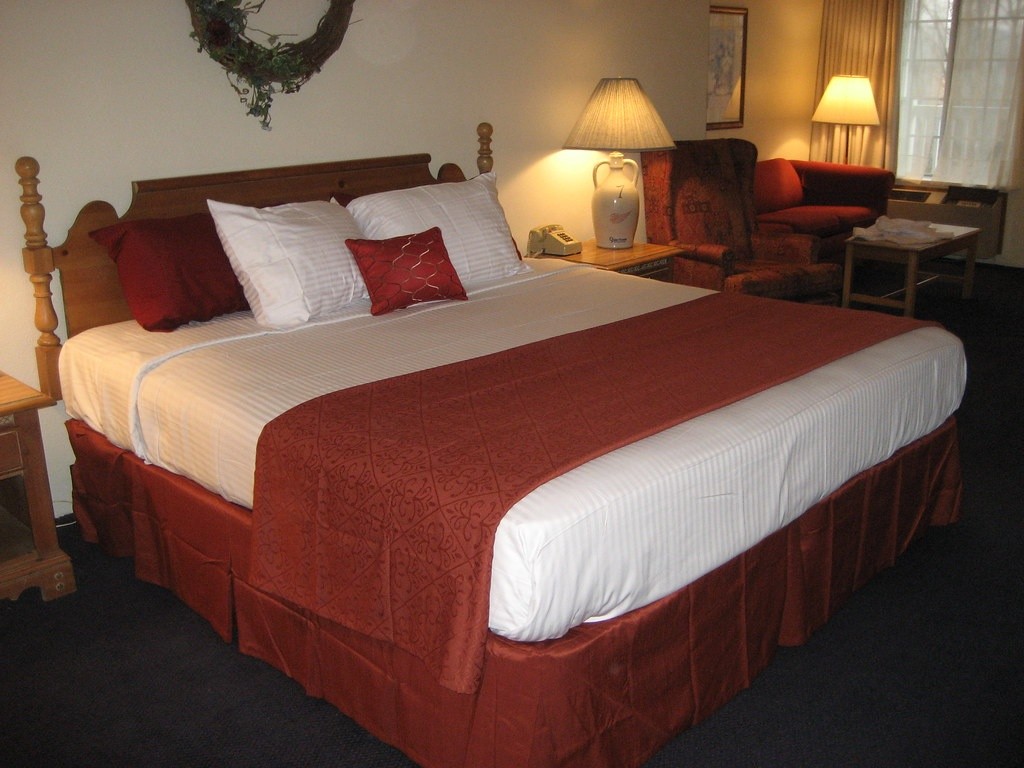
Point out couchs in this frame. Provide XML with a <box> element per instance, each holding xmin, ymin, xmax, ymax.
<box><xmin>640</xmin><ymin>138</ymin><xmax>843</xmax><ymax>305</ymax></box>
<box><xmin>753</xmin><ymin>160</ymin><xmax>895</xmax><ymax>275</ymax></box>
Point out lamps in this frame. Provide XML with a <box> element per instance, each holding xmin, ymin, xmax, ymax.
<box><xmin>812</xmin><ymin>75</ymin><xmax>880</xmax><ymax>163</ymax></box>
<box><xmin>563</xmin><ymin>78</ymin><xmax>678</xmax><ymax>250</ymax></box>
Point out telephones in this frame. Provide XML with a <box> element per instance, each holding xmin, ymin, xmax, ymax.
<box><xmin>527</xmin><ymin>224</ymin><xmax>582</xmax><ymax>256</ymax></box>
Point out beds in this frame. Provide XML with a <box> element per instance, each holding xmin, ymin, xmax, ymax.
<box><xmin>13</xmin><ymin>121</ymin><xmax>969</xmax><ymax>768</ymax></box>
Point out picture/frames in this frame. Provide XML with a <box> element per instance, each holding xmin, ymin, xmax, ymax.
<box><xmin>706</xmin><ymin>6</ymin><xmax>748</xmax><ymax>129</ymax></box>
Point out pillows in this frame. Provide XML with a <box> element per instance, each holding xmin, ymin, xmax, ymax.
<box><xmin>87</xmin><ymin>214</ymin><xmax>251</xmax><ymax>333</ymax></box>
<box><xmin>346</xmin><ymin>226</ymin><xmax>469</xmax><ymax>317</ymax></box>
<box><xmin>346</xmin><ymin>170</ymin><xmax>534</xmax><ymax>287</ymax></box>
<box><xmin>754</xmin><ymin>158</ymin><xmax>803</xmax><ymax>215</ymax></box>
<box><xmin>206</xmin><ymin>196</ymin><xmax>370</xmax><ymax>328</ymax></box>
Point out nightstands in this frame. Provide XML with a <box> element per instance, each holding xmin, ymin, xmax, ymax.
<box><xmin>525</xmin><ymin>239</ymin><xmax>685</xmax><ymax>282</ymax></box>
<box><xmin>0</xmin><ymin>370</ymin><xmax>77</xmax><ymax>603</ymax></box>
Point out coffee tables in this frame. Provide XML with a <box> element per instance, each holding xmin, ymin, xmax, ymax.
<box><xmin>843</xmin><ymin>219</ymin><xmax>981</xmax><ymax>320</ymax></box>
